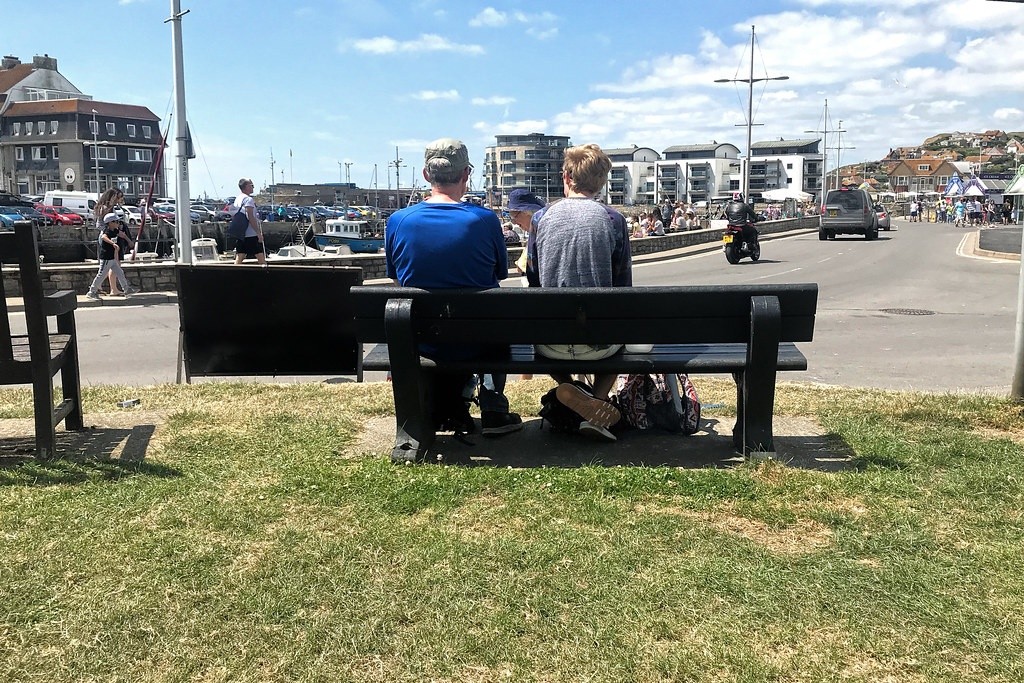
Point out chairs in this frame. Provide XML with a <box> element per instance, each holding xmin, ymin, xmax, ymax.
<box><xmin>0</xmin><ymin>221</ymin><xmax>84</xmax><ymax>464</ymax></box>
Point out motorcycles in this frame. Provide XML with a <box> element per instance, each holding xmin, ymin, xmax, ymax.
<box><xmin>723</xmin><ymin>216</ymin><xmax>761</xmax><ymax>265</ymax></box>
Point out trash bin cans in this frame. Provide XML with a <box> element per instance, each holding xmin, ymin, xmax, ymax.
<box><xmin>1019</xmin><ymin>210</ymin><xmax>1024</xmax><ymax>221</ymax></box>
<box><xmin>699</xmin><ymin>220</ymin><xmax>711</xmax><ymax>229</ymax></box>
<box><xmin>993</xmin><ymin>204</ymin><xmax>1003</xmax><ymax>222</ymax></box>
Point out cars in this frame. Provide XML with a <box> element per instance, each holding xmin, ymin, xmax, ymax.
<box><xmin>873</xmin><ymin>205</ymin><xmax>890</xmax><ymax>231</ymax></box>
<box><xmin>0</xmin><ymin>187</ymin><xmax>362</xmax><ymax>229</ymax></box>
<box><xmin>818</xmin><ymin>190</ymin><xmax>879</xmax><ymax>241</ymax></box>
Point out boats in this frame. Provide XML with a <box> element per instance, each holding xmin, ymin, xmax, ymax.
<box><xmin>312</xmin><ymin>215</ymin><xmax>384</xmax><ymax>255</ymax></box>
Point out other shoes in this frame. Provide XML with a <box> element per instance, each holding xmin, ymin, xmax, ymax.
<box><xmin>955</xmin><ymin>225</ymin><xmax>966</xmax><ymax>227</ymax></box>
<box><xmin>752</xmin><ymin>244</ymin><xmax>758</xmax><ymax>250</ymax></box>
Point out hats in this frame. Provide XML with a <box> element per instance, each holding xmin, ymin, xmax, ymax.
<box><xmin>103</xmin><ymin>213</ymin><xmax>123</xmax><ymax>223</ymax></box>
<box><xmin>665</xmin><ymin>199</ymin><xmax>669</xmax><ymax>202</ymax></box>
<box><xmin>960</xmin><ymin>198</ymin><xmax>963</xmax><ymax>200</ymax></box>
<box><xmin>508</xmin><ymin>189</ymin><xmax>545</xmax><ymax>210</ymax></box>
<box><xmin>425</xmin><ymin>138</ymin><xmax>474</xmax><ymax>173</ymax></box>
<box><xmin>686</xmin><ymin>211</ymin><xmax>692</xmax><ymax>214</ymax></box>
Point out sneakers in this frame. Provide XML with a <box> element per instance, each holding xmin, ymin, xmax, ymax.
<box><xmin>451</xmin><ymin>416</ymin><xmax>475</xmax><ymax>436</ymax></box>
<box><xmin>556</xmin><ymin>380</ymin><xmax>621</xmax><ymax>441</ymax></box>
<box><xmin>481</xmin><ymin>411</ymin><xmax>523</xmax><ymax>437</ymax></box>
<box><xmin>124</xmin><ymin>288</ymin><xmax>140</xmax><ymax>298</ymax></box>
<box><xmin>85</xmin><ymin>292</ymin><xmax>102</xmax><ymax>300</ymax></box>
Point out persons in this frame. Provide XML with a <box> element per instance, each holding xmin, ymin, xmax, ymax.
<box><xmin>630</xmin><ymin>192</ymin><xmax>821</xmax><ymax>252</ymax></box>
<box><xmin>503</xmin><ymin>222</ymin><xmax>520</xmax><ymax>242</ymax></box>
<box><xmin>505</xmin><ymin>187</ymin><xmax>595</xmax><ymax>388</ymax></box>
<box><xmin>85</xmin><ymin>186</ymin><xmax>141</xmax><ymax>301</ymax></box>
<box><xmin>908</xmin><ymin>197</ymin><xmax>1017</xmax><ymax>227</ymax></box>
<box><xmin>234</xmin><ymin>177</ymin><xmax>264</xmax><ymax>266</ymax></box>
<box><xmin>385</xmin><ymin>138</ymin><xmax>523</xmax><ymax>438</ymax></box>
<box><xmin>526</xmin><ymin>144</ymin><xmax>634</xmax><ymax>443</ymax></box>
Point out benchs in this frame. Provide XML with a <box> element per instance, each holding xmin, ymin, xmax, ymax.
<box><xmin>351</xmin><ymin>281</ymin><xmax>819</xmax><ymax>467</ymax></box>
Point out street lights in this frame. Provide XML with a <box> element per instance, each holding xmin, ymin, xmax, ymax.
<box><xmin>712</xmin><ymin>75</ymin><xmax>790</xmax><ymax>219</ymax></box>
<box><xmin>804</xmin><ymin>129</ymin><xmax>846</xmax><ymax>226</ymax></box>
<box><xmin>388</xmin><ymin>164</ymin><xmax>408</xmax><ymax>210</ymax></box>
<box><xmin>824</xmin><ymin>144</ymin><xmax>855</xmax><ymax>189</ymax></box>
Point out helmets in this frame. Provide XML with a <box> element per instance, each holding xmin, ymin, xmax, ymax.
<box><xmin>733</xmin><ymin>191</ymin><xmax>746</xmax><ymax>203</ymax></box>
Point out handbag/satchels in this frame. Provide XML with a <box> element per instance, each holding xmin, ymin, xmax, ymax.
<box><xmin>538</xmin><ymin>381</ymin><xmax>611</xmax><ymax>430</ymax></box>
<box><xmin>223</xmin><ymin>197</ymin><xmax>249</xmax><ymax>241</ymax></box>
<box><xmin>1003</xmin><ymin>207</ymin><xmax>1006</xmax><ymax>212</ymax></box>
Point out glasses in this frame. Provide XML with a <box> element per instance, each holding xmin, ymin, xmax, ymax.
<box><xmin>465</xmin><ymin>166</ymin><xmax>472</xmax><ymax>174</ymax></box>
<box><xmin>248</xmin><ymin>184</ymin><xmax>254</xmax><ymax>187</ymax></box>
<box><xmin>559</xmin><ymin>171</ymin><xmax>573</xmax><ymax>179</ymax></box>
<box><xmin>511</xmin><ymin>211</ymin><xmax>521</xmax><ymax>224</ymax></box>
<box><xmin>689</xmin><ymin>215</ymin><xmax>691</xmax><ymax>217</ymax></box>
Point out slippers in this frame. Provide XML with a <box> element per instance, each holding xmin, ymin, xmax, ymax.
<box><xmin>110</xmin><ymin>292</ymin><xmax>124</xmax><ymax>296</ymax></box>
<box><xmin>97</xmin><ymin>290</ymin><xmax>107</xmax><ymax>295</ymax></box>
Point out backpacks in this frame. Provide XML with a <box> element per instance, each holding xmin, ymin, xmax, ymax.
<box><xmin>615</xmin><ymin>374</ymin><xmax>700</xmax><ymax>436</ymax></box>
